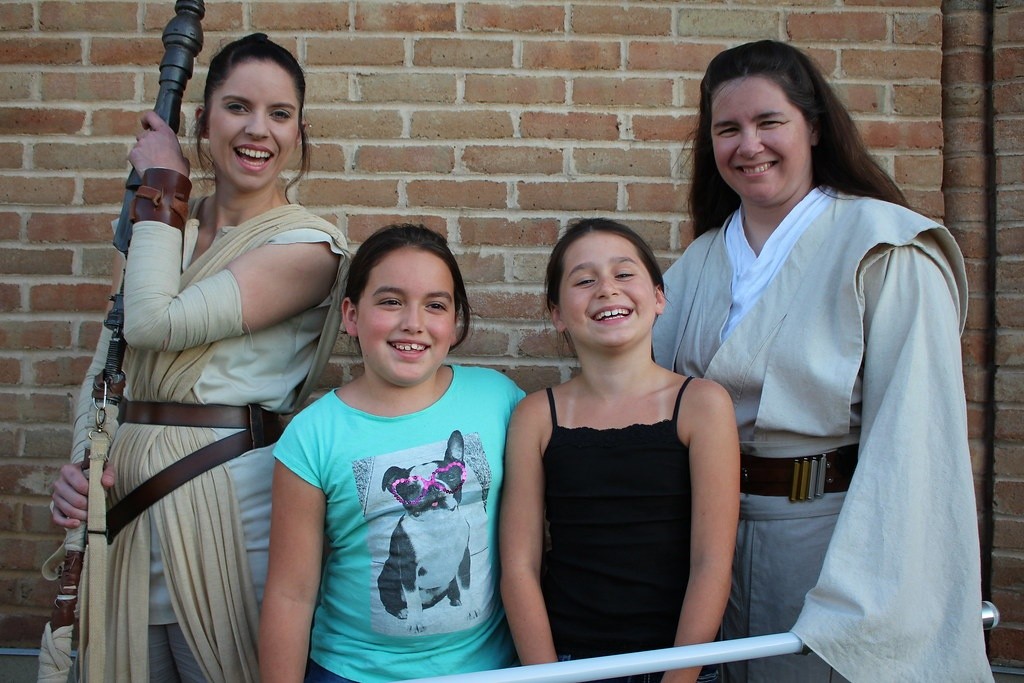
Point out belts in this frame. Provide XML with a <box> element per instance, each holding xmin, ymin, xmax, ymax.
<box><xmin>82</xmin><ymin>399</ymin><xmax>279</xmax><ymax>547</ymax></box>
<box><xmin>740</xmin><ymin>443</ymin><xmax>861</xmax><ymax>502</ymax></box>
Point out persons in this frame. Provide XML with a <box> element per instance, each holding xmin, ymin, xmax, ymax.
<box><xmin>651</xmin><ymin>39</ymin><xmax>998</xmax><ymax>683</ymax></box>
<box><xmin>259</xmin><ymin>224</ymin><xmax>525</xmax><ymax>683</ymax></box>
<box><xmin>500</xmin><ymin>217</ymin><xmax>739</xmax><ymax>683</ymax></box>
<box><xmin>49</xmin><ymin>33</ymin><xmax>349</xmax><ymax>682</ymax></box>
